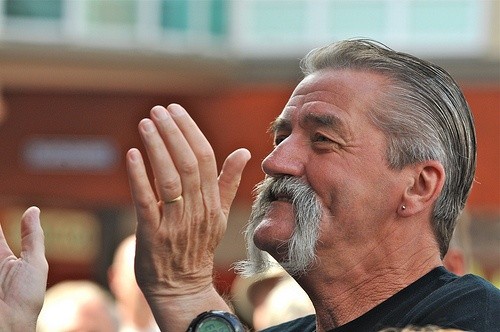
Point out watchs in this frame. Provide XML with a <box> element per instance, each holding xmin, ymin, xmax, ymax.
<box><xmin>186</xmin><ymin>309</ymin><xmax>245</xmax><ymax>332</ymax></box>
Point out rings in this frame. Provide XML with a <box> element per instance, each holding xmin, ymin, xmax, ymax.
<box><xmin>161</xmin><ymin>194</ymin><xmax>185</xmax><ymax>204</ymax></box>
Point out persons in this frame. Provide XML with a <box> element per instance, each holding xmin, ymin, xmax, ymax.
<box><xmin>0</xmin><ymin>37</ymin><xmax>500</xmax><ymax>332</ymax></box>
<box><xmin>30</xmin><ymin>280</ymin><xmax>119</xmax><ymax>332</ymax></box>
<box><xmin>246</xmin><ymin>266</ymin><xmax>318</xmax><ymax>330</ymax></box>
<box><xmin>107</xmin><ymin>230</ymin><xmax>165</xmax><ymax>332</ymax></box>
